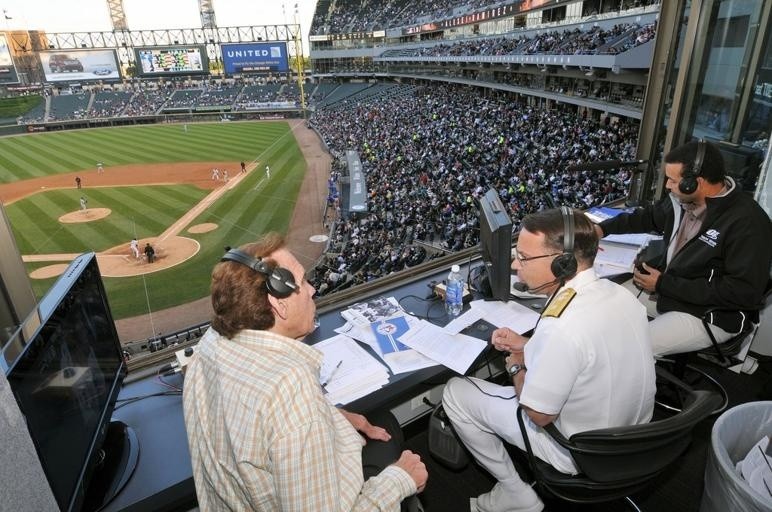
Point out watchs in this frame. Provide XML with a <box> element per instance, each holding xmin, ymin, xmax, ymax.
<box><xmin>509</xmin><ymin>362</ymin><xmax>527</xmax><ymax>376</ymax></box>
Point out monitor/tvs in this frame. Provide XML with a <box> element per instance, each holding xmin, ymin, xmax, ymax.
<box><xmin>0</xmin><ymin>251</ymin><xmax>140</xmax><ymax>512</ymax></box>
<box><xmin>474</xmin><ymin>187</ymin><xmax>516</xmax><ymax>303</ymax></box>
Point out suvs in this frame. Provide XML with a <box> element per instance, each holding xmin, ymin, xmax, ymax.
<box><xmin>49</xmin><ymin>55</ymin><xmax>84</xmax><ymax>73</ymax></box>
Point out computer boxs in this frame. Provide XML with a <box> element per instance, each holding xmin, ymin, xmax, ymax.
<box><xmin>430</xmin><ymin>404</ymin><xmax>472</xmax><ymax>472</ymax></box>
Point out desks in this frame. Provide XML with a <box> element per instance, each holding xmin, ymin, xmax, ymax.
<box><xmin>100</xmin><ymin>200</ymin><xmax>665</xmax><ymax>512</ymax></box>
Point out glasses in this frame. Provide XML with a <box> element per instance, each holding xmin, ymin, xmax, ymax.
<box><xmin>515</xmin><ymin>253</ymin><xmax>562</xmax><ymax>266</ymax></box>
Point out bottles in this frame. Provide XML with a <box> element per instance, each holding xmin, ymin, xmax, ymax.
<box><xmin>444</xmin><ymin>265</ymin><xmax>464</xmax><ymax>317</ymax></box>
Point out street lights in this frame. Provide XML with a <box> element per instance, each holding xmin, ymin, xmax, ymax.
<box><xmin>283</xmin><ymin>3</ymin><xmax>305</xmax><ymax>108</ymax></box>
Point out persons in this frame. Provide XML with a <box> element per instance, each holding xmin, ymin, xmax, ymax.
<box><xmin>440</xmin><ymin>207</ymin><xmax>658</xmax><ymax>512</ymax></box>
<box><xmin>594</xmin><ymin>138</ymin><xmax>772</xmax><ymax>367</ymax></box>
<box><xmin>122</xmin><ymin>330</ymin><xmax>202</xmax><ymax>361</ymax></box>
<box><xmin>0</xmin><ymin>3</ymin><xmax>772</xmax><ymax>298</ymax></box>
<box><xmin>182</xmin><ymin>230</ymin><xmax>430</xmax><ymax>511</ymax></box>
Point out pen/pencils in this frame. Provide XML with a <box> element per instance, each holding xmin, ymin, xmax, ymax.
<box><xmin>323</xmin><ymin>361</ymin><xmax>343</xmax><ymax>387</ymax></box>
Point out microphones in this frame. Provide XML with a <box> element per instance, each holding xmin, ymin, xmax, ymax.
<box><xmin>513</xmin><ymin>273</ymin><xmax>566</xmax><ymax>292</ymax></box>
<box><xmin>570</xmin><ymin>160</ymin><xmax>648</xmax><ymax>171</ymax></box>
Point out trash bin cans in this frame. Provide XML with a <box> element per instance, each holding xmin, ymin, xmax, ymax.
<box><xmin>699</xmin><ymin>399</ymin><xmax>772</xmax><ymax>512</ymax></box>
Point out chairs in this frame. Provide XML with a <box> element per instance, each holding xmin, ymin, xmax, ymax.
<box><xmin>696</xmin><ymin>277</ymin><xmax>772</xmax><ymax>367</ymax></box>
<box><xmin>501</xmin><ymin>356</ymin><xmax>730</xmax><ymax>512</ymax></box>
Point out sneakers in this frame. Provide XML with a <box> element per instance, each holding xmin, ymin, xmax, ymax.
<box><xmin>476</xmin><ymin>482</ymin><xmax>545</xmax><ymax>512</ymax></box>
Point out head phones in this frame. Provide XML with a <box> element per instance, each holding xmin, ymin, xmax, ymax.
<box><xmin>679</xmin><ymin>137</ymin><xmax>707</xmax><ymax>194</ymax></box>
<box><xmin>221</xmin><ymin>248</ymin><xmax>300</xmax><ymax>299</ymax></box>
<box><xmin>551</xmin><ymin>206</ymin><xmax>577</xmax><ymax>280</ymax></box>
